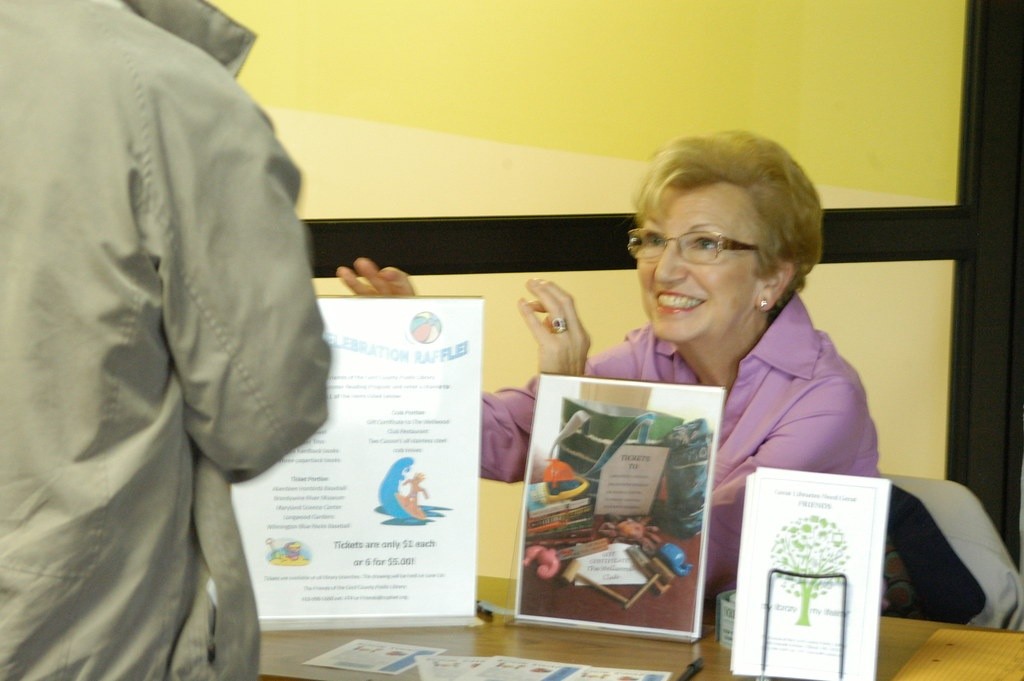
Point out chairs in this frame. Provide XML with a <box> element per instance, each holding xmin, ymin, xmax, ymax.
<box><xmin>884</xmin><ymin>474</ymin><xmax>1024</xmax><ymax>634</ymax></box>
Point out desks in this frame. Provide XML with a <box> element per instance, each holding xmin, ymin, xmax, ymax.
<box><xmin>258</xmin><ymin>575</ymin><xmax>1024</xmax><ymax>681</ymax></box>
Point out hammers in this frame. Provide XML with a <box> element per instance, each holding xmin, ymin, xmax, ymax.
<box><xmin>624</xmin><ymin>557</ymin><xmax>676</xmax><ymax>610</ymax></box>
<box><xmin>561</xmin><ymin>560</ymin><xmax>629</xmax><ymax>604</ymax></box>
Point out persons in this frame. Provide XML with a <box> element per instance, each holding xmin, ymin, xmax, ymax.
<box><xmin>336</xmin><ymin>131</ymin><xmax>891</xmax><ymax>621</ymax></box>
<box><xmin>0</xmin><ymin>0</ymin><xmax>332</xmax><ymax>681</ymax></box>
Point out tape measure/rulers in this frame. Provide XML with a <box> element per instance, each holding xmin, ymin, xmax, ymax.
<box><xmin>556</xmin><ymin>538</ymin><xmax>609</xmax><ymax>562</ymax></box>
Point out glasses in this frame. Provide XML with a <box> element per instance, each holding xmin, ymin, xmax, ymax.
<box><xmin>627</xmin><ymin>227</ymin><xmax>759</xmax><ymax>265</ymax></box>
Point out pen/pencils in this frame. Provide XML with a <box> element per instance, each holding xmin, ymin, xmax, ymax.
<box><xmin>675</xmin><ymin>657</ymin><xmax>704</xmax><ymax>681</ymax></box>
<box><xmin>476</xmin><ymin>604</ymin><xmax>494</xmax><ymax>619</ymax></box>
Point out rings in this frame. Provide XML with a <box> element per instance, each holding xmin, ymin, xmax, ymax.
<box><xmin>551</xmin><ymin>318</ymin><xmax>565</xmax><ymax>333</ymax></box>
